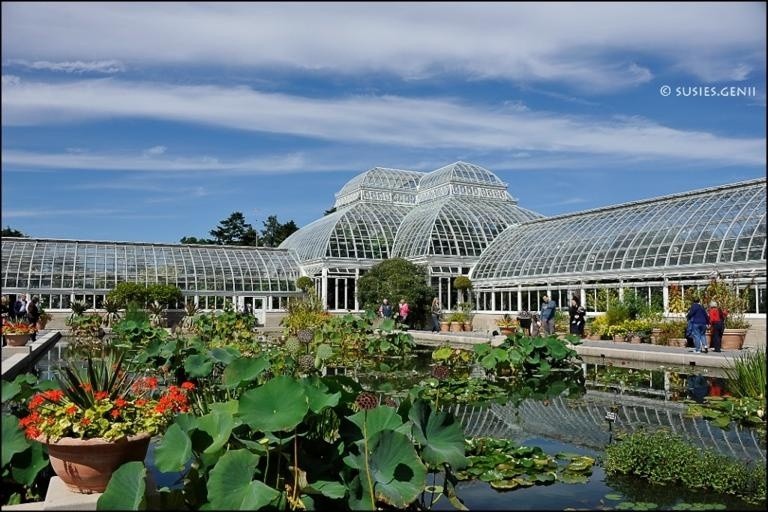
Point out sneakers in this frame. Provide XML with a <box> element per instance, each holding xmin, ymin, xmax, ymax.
<box><xmin>694</xmin><ymin>345</ymin><xmax>708</xmax><ymax>354</ymax></box>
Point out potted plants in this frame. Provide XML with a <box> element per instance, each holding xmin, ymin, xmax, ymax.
<box><xmin>651</xmin><ymin>272</ymin><xmax>752</xmax><ymax>350</ymax></box>
<box><xmin>439</xmin><ymin>303</ymin><xmax>474</xmax><ymax>332</ymax></box>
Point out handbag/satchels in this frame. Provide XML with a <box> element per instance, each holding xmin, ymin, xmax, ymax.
<box><xmin>718</xmin><ymin>308</ymin><xmax>724</xmax><ymax>321</ymax></box>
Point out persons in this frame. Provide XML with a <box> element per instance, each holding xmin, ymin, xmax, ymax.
<box><xmin>515</xmin><ymin>294</ymin><xmax>585</xmax><ymax>338</ymax></box>
<box><xmin>379</xmin><ymin>298</ymin><xmax>393</xmax><ymax>318</ymax></box>
<box><xmin>686</xmin><ymin>298</ymin><xmax>708</xmax><ymax>353</ymax></box>
<box><xmin>431</xmin><ymin>297</ymin><xmax>442</xmax><ymax>334</ymax></box>
<box><xmin>689</xmin><ymin>375</ymin><xmax>734</xmax><ymax>420</ymax></box>
<box><xmin>2</xmin><ymin>293</ymin><xmax>40</xmax><ymax>342</ymax></box>
<box><xmin>398</xmin><ymin>299</ymin><xmax>410</xmax><ymax>331</ymax></box>
<box><xmin>708</xmin><ymin>301</ymin><xmax>727</xmax><ymax>352</ymax></box>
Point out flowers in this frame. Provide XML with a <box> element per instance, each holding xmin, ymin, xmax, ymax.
<box><xmin>495</xmin><ymin>319</ymin><xmax>520</xmax><ymax>326</ymax></box>
<box><xmin>589</xmin><ymin>315</ymin><xmax>646</xmax><ymax>337</ymax></box>
<box><xmin>17</xmin><ymin>348</ymin><xmax>196</xmax><ymax>443</ymax></box>
<box><xmin>1</xmin><ymin>320</ymin><xmax>38</xmax><ymax>337</ymax></box>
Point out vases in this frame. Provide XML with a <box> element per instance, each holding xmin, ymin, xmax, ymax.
<box><xmin>32</xmin><ymin>432</ymin><xmax>151</xmax><ymax>494</ymax></box>
<box><xmin>613</xmin><ymin>335</ymin><xmax>642</xmax><ymax>344</ymax></box>
<box><xmin>591</xmin><ymin>335</ymin><xmax>601</xmax><ymax>340</ymax></box>
<box><xmin>499</xmin><ymin>326</ymin><xmax>516</xmax><ymax>335</ymax></box>
<box><xmin>4</xmin><ymin>332</ymin><xmax>29</xmax><ymax>345</ymax></box>
